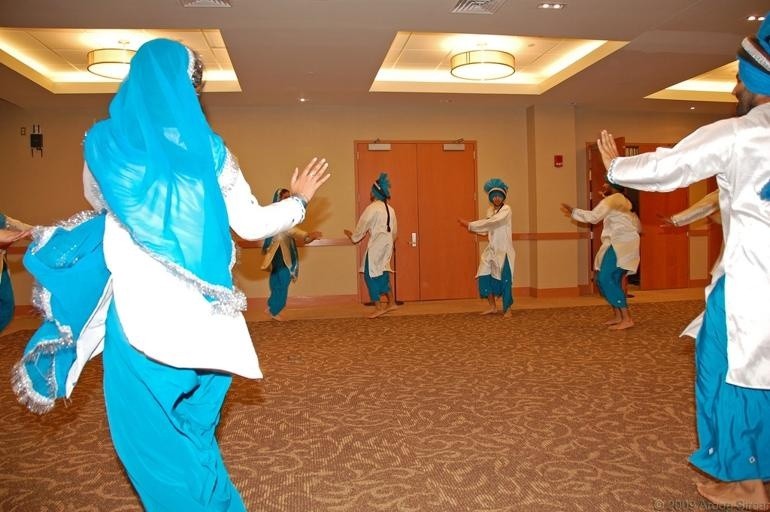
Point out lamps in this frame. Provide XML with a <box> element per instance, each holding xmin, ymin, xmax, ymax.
<box><xmin>449</xmin><ymin>48</ymin><xmax>515</xmax><ymax>81</ymax></box>
<box><xmin>86</xmin><ymin>47</ymin><xmax>139</xmax><ymax>80</ymax></box>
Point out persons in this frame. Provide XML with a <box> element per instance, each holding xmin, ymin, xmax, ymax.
<box><xmin>457</xmin><ymin>177</ymin><xmax>516</xmax><ymax>320</ymax></box>
<box><xmin>9</xmin><ymin>36</ymin><xmax>331</xmax><ymax>508</ymax></box>
<box><xmin>656</xmin><ymin>188</ymin><xmax>724</xmax><ymax>279</ymax></box>
<box><xmin>597</xmin><ymin>8</ymin><xmax>770</xmax><ymax>511</ymax></box>
<box><xmin>0</xmin><ymin>213</ymin><xmax>38</xmax><ymax>336</ymax></box>
<box><xmin>560</xmin><ymin>171</ymin><xmax>641</xmax><ymax>331</ymax></box>
<box><xmin>621</xmin><ymin>200</ymin><xmax>642</xmax><ymax>299</ymax></box>
<box><xmin>261</xmin><ymin>188</ymin><xmax>322</xmax><ymax>321</ymax></box>
<box><xmin>344</xmin><ymin>172</ymin><xmax>398</xmax><ymax>319</ymax></box>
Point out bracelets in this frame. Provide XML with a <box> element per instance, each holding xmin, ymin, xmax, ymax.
<box><xmin>292</xmin><ymin>191</ymin><xmax>310</xmax><ymax>208</ymax></box>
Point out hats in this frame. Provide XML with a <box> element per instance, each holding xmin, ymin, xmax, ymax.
<box><xmin>371</xmin><ymin>172</ymin><xmax>391</xmax><ymax>201</ymax></box>
<box><xmin>483</xmin><ymin>179</ymin><xmax>508</xmax><ymax>201</ymax></box>
<box><xmin>736</xmin><ymin>13</ymin><xmax>769</xmax><ymax>98</ymax></box>
